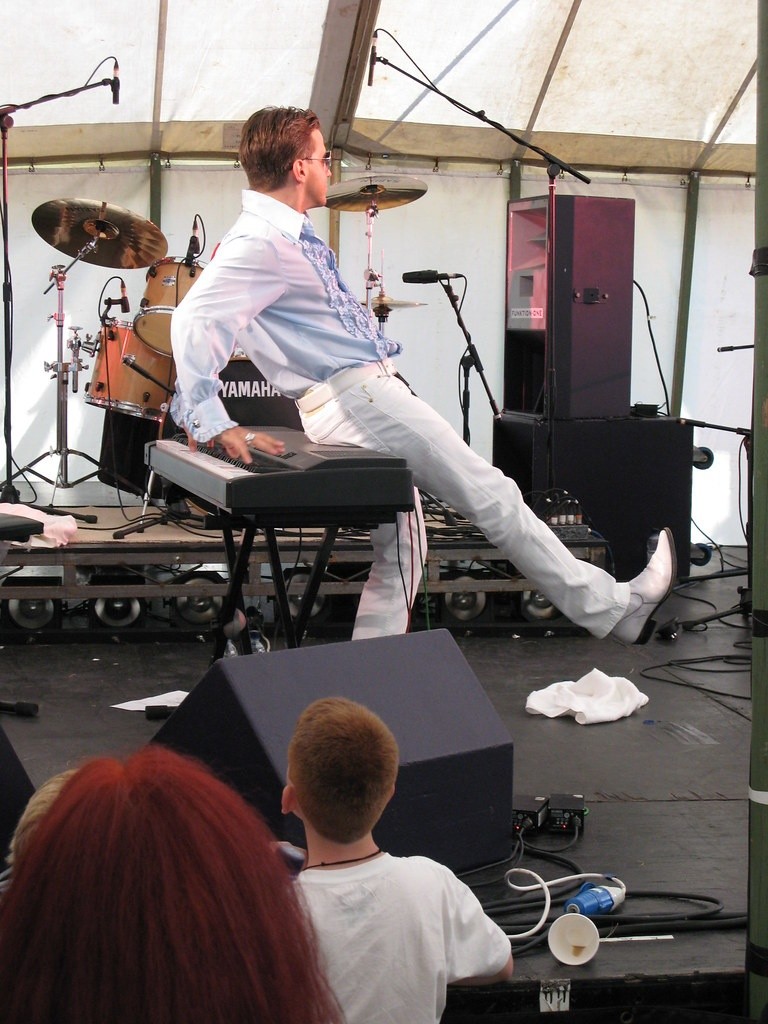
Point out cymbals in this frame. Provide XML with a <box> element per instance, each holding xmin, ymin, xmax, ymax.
<box><xmin>31</xmin><ymin>197</ymin><xmax>169</xmax><ymax>270</ymax></box>
<box><xmin>320</xmin><ymin>174</ymin><xmax>429</xmax><ymax>211</ymax></box>
<box><xmin>132</xmin><ymin>257</ymin><xmax>232</xmax><ymax>359</ymax></box>
<box><xmin>359</xmin><ymin>293</ymin><xmax>430</xmax><ymax>315</ymax></box>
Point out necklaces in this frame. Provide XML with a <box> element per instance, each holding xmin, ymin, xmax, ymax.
<box><xmin>303</xmin><ymin>848</ymin><xmax>386</xmax><ymax>869</ymax></box>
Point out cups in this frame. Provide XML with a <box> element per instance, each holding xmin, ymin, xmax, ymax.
<box><xmin>548</xmin><ymin>914</ymin><xmax>599</xmax><ymax>966</ymax></box>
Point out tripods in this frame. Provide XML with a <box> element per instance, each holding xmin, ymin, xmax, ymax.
<box><xmin>0</xmin><ymin>231</ymin><xmax>159</xmax><ymax>507</ymax></box>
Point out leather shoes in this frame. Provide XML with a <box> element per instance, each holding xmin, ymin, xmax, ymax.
<box><xmin>610</xmin><ymin>525</ymin><xmax>677</xmax><ymax>648</ymax></box>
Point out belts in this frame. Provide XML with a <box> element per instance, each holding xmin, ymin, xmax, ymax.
<box><xmin>293</xmin><ymin>356</ymin><xmax>396</xmax><ymax>413</ymax></box>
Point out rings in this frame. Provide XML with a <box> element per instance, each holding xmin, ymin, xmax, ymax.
<box><xmin>244</xmin><ymin>432</ymin><xmax>255</xmax><ymax>443</ymax></box>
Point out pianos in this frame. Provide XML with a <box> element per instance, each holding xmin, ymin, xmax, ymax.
<box><xmin>141</xmin><ymin>424</ymin><xmax>416</xmax><ymax>720</ymax></box>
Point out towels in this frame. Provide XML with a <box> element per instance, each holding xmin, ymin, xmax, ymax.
<box><xmin>522</xmin><ymin>666</ymin><xmax>651</xmax><ymax>726</ymax></box>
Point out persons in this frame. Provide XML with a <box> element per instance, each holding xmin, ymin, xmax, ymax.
<box><xmin>275</xmin><ymin>695</ymin><xmax>514</xmax><ymax>1024</ymax></box>
<box><xmin>1</xmin><ymin>748</ymin><xmax>348</xmax><ymax>1022</ymax></box>
<box><xmin>171</xmin><ymin>110</ymin><xmax>680</xmax><ymax>648</ymax></box>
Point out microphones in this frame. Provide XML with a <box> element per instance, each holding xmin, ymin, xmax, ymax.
<box><xmin>368</xmin><ymin>31</ymin><xmax>377</xmax><ymax>86</ymax></box>
<box><xmin>121</xmin><ymin>281</ymin><xmax>129</xmax><ymax>313</ymax></box>
<box><xmin>192</xmin><ymin>220</ymin><xmax>200</xmax><ymax>266</ymax></box>
<box><xmin>113</xmin><ymin>61</ymin><xmax>120</xmax><ymax>104</ymax></box>
<box><xmin>403</xmin><ymin>270</ymin><xmax>461</xmax><ymax>283</ymax></box>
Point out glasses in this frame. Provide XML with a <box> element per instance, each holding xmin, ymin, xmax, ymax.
<box><xmin>290</xmin><ymin>150</ymin><xmax>332</xmax><ymax>167</ymax></box>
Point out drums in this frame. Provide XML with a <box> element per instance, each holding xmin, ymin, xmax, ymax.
<box><xmin>84</xmin><ymin>320</ymin><xmax>177</xmax><ymax>423</ymax></box>
<box><xmin>158</xmin><ymin>357</ymin><xmax>305</xmax><ymax>516</ymax></box>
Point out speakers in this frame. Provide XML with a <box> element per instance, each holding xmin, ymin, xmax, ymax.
<box><xmin>148</xmin><ymin>627</ymin><xmax>513</xmax><ymax>872</ymax></box>
<box><xmin>493</xmin><ymin>414</ymin><xmax>694</xmax><ymax>583</ymax></box>
<box><xmin>503</xmin><ymin>195</ymin><xmax>635</xmax><ymax>420</ymax></box>
